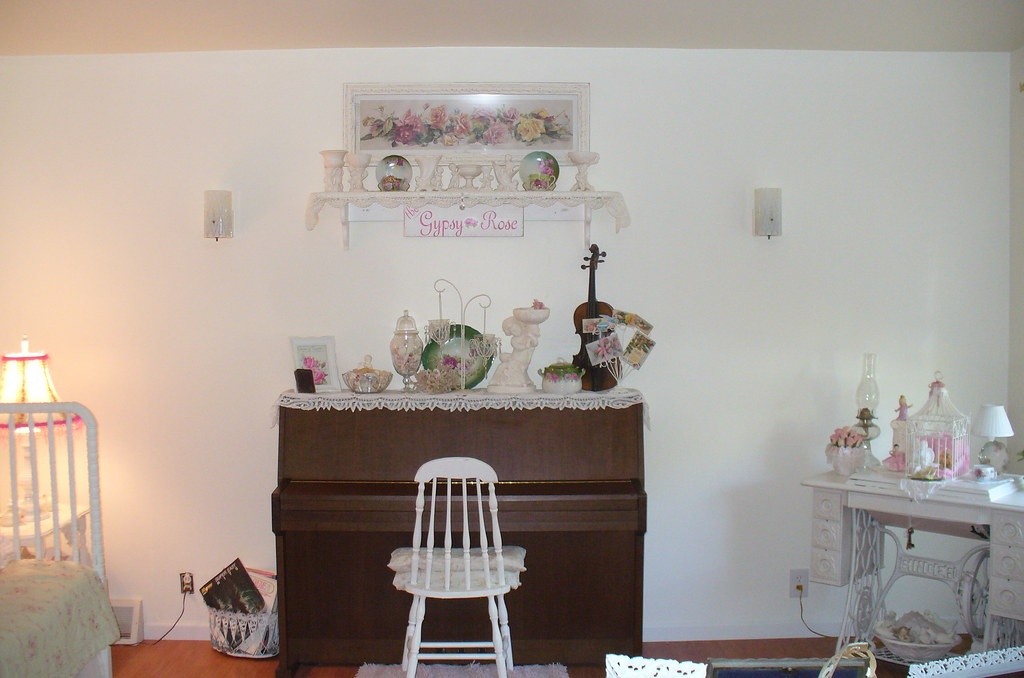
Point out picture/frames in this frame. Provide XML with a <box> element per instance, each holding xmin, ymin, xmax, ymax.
<box><xmin>290</xmin><ymin>336</ymin><xmax>341</xmax><ymax>392</ymax></box>
<box><xmin>341</xmin><ymin>81</ymin><xmax>589</xmax><ymax>168</ymax></box>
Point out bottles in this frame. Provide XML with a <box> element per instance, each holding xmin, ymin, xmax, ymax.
<box><xmin>536</xmin><ymin>359</ymin><xmax>585</xmax><ymax>394</ymax></box>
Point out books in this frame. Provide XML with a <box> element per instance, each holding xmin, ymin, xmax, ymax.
<box><xmin>199</xmin><ymin>558</ymin><xmax>277</xmax><ymax>656</ymax></box>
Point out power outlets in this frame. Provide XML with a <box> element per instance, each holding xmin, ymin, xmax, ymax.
<box><xmin>181</xmin><ymin>573</ymin><xmax>194</xmax><ymax>594</ymax></box>
<box><xmin>791</xmin><ymin>568</ymin><xmax>809</xmax><ymax>597</ymax></box>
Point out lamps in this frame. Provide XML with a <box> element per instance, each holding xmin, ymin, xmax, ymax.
<box><xmin>753</xmin><ymin>187</ymin><xmax>781</xmax><ymax>239</ymax></box>
<box><xmin>853</xmin><ymin>353</ymin><xmax>882</xmax><ymax>469</ymax></box>
<box><xmin>972</xmin><ymin>404</ymin><xmax>1015</xmax><ymax>442</ymax></box>
<box><xmin>0</xmin><ymin>338</ymin><xmax>66</xmax><ymax>527</ymax></box>
<box><xmin>203</xmin><ymin>190</ymin><xmax>233</xmax><ymax>240</ymax></box>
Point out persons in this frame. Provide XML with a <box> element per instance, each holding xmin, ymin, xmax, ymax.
<box><xmin>895</xmin><ymin>395</ymin><xmax>913</xmax><ymax>421</ymax></box>
<box><xmin>491</xmin><ymin>316</ymin><xmax>539</xmax><ymax>385</ymax></box>
<box><xmin>882</xmin><ymin>445</ymin><xmax>906</xmax><ymax>472</ymax></box>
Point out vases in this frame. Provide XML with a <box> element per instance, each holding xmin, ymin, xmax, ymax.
<box><xmin>824</xmin><ymin>444</ymin><xmax>863</xmax><ymax>477</ymax></box>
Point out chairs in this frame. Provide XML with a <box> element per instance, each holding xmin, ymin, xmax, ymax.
<box><xmin>390</xmin><ymin>457</ymin><xmax>525</xmax><ymax>678</ymax></box>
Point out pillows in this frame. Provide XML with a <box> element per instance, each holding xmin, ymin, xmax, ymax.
<box><xmin>392</xmin><ymin>570</ymin><xmax>522</xmax><ymax>589</ymax></box>
<box><xmin>386</xmin><ymin>547</ymin><xmax>528</xmax><ymax>573</ymax></box>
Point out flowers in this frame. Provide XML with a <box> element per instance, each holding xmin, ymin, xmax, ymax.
<box><xmin>359</xmin><ymin>99</ymin><xmax>572</xmax><ymax>146</ymax></box>
<box><xmin>829</xmin><ymin>426</ymin><xmax>865</xmax><ymax>447</ymax></box>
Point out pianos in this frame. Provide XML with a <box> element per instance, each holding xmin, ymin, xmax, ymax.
<box><xmin>270</xmin><ymin>388</ymin><xmax>650</xmax><ymax>677</ymax></box>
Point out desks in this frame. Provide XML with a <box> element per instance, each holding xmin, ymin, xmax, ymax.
<box><xmin>802</xmin><ymin>475</ymin><xmax>1023</xmax><ymax>623</ymax></box>
<box><xmin>3</xmin><ymin>504</ymin><xmax>92</xmax><ymax>568</ymax></box>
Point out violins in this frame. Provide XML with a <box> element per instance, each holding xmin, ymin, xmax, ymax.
<box><xmin>572</xmin><ymin>242</ymin><xmax>622</xmax><ymax>391</ymax></box>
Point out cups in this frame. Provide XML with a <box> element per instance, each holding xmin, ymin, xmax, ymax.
<box><xmin>972</xmin><ymin>464</ymin><xmax>997</xmax><ymax>481</ymax></box>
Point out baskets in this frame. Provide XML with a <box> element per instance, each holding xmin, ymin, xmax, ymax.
<box><xmin>205</xmin><ymin>603</ymin><xmax>281</xmax><ymax>658</ymax></box>
<box><xmin>873</xmin><ymin>631</ymin><xmax>962</xmax><ymax>662</ymax></box>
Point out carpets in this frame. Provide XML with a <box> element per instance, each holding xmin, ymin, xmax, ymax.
<box><xmin>355</xmin><ymin>662</ymin><xmax>570</xmax><ymax>678</ymax></box>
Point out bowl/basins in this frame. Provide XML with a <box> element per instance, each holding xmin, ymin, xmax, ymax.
<box><xmin>342</xmin><ymin>370</ymin><xmax>393</xmax><ymax>393</ymax></box>
<box><xmin>872</xmin><ymin>630</ymin><xmax>962</xmax><ymax>662</ymax></box>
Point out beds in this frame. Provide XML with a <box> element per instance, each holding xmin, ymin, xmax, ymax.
<box><xmin>0</xmin><ymin>402</ymin><xmax>115</xmax><ymax>677</ymax></box>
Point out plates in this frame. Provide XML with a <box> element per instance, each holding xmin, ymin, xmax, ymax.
<box><xmin>519</xmin><ymin>151</ymin><xmax>559</xmax><ymax>189</ymax></box>
<box><xmin>422</xmin><ymin>324</ymin><xmax>494</xmax><ymax>390</ymax></box>
<box><xmin>375</xmin><ymin>154</ymin><xmax>413</xmax><ymax>188</ymax></box>
